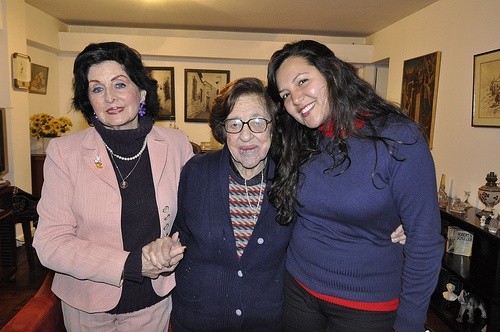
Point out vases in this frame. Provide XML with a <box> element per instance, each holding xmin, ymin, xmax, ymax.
<box><xmin>38</xmin><ymin>138</ymin><xmax>52</xmax><ymax>151</ymax></box>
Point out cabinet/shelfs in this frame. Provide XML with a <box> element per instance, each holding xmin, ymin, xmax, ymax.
<box><xmin>423</xmin><ymin>196</ymin><xmax>500</xmax><ymax>332</ymax></box>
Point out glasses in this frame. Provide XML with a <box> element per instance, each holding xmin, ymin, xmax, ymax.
<box><xmin>221</xmin><ymin>118</ymin><xmax>273</xmax><ymax>134</ymax></box>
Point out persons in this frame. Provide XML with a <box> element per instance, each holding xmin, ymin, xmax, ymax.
<box><xmin>261</xmin><ymin>40</ymin><xmax>443</xmax><ymax>332</ymax></box>
<box><xmin>33</xmin><ymin>42</ymin><xmax>196</xmax><ymax>332</ymax></box>
<box><xmin>443</xmin><ymin>284</ymin><xmax>457</xmax><ymax>301</ymax></box>
<box><xmin>141</xmin><ymin>77</ymin><xmax>405</xmax><ymax>332</ymax></box>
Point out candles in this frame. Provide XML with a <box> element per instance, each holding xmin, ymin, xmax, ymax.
<box><xmin>449</xmin><ymin>179</ymin><xmax>453</xmax><ymax>197</ymax></box>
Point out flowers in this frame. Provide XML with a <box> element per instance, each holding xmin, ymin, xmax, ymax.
<box><xmin>29</xmin><ymin>112</ymin><xmax>73</xmax><ymax>143</ymax></box>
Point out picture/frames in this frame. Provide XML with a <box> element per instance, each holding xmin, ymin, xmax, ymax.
<box><xmin>471</xmin><ymin>49</ymin><xmax>500</xmax><ymax>128</ymax></box>
<box><xmin>401</xmin><ymin>51</ymin><xmax>441</xmax><ymax>150</ymax></box>
<box><xmin>144</xmin><ymin>67</ymin><xmax>175</xmax><ymax>121</ymax></box>
<box><xmin>0</xmin><ymin>108</ymin><xmax>9</xmax><ymax>178</ymax></box>
<box><xmin>29</xmin><ymin>63</ymin><xmax>49</xmax><ymax>95</ymax></box>
<box><xmin>184</xmin><ymin>68</ymin><xmax>230</xmax><ymax>122</ymax></box>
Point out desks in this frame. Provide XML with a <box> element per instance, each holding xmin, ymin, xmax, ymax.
<box><xmin>31</xmin><ymin>149</ymin><xmax>47</xmax><ymax>228</ymax></box>
<box><xmin>0</xmin><ymin>186</ymin><xmax>38</xmax><ymax>288</ymax></box>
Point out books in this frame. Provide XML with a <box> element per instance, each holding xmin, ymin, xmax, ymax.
<box><xmin>446</xmin><ymin>227</ymin><xmax>473</xmax><ymax>256</ymax></box>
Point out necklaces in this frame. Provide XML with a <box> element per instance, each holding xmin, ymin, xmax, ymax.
<box><xmin>104</xmin><ymin>140</ymin><xmax>147</xmax><ymax>190</ymax></box>
<box><xmin>244</xmin><ymin>167</ymin><xmax>263</xmax><ymax>224</ymax></box>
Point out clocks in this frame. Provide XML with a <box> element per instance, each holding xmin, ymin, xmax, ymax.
<box><xmin>12</xmin><ymin>52</ymin><xmax>31</xmax><ymax>89</ymax></box>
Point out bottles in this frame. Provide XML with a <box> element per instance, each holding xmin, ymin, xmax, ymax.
<box><xmin>437</xmin><ymin>174</ymin><xmax>448</xmax><ymax>208</ymax></box>
